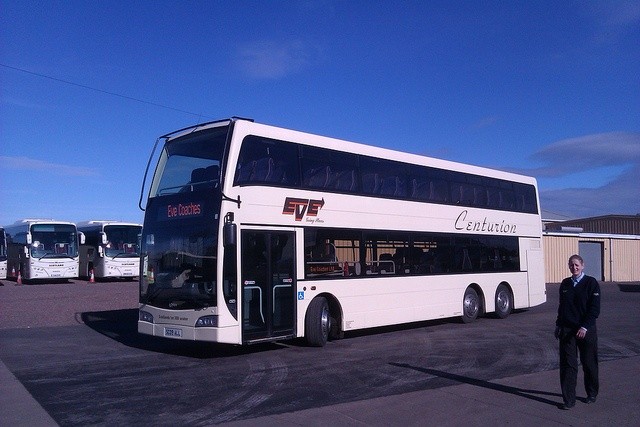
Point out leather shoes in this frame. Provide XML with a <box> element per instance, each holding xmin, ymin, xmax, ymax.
<box><xmin>564</xmin><ymin>401</ymin><xmax>575</xmax><ymax>409</ymax></box>
<box><xmin>587</xmin><ymin>395</ymin><xmax>595</xmax><ymax>403</ymax></box>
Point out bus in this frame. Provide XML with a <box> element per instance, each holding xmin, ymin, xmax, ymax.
<box><xmin>5</xmin><ymin>218</ymin><xmax>85</xmax><ymax>280</ymax></box>
<box><xmin>0</xmin><ymin>227</ymin><xmax>8</xmax><ymax>279</ymax></box>
<box><xmin>77</xmin><ymin>220</ymin><xmax>148</xmax><ymax>280</ymax></box>
<box><xmin>137</xmin><ymin>116</ymin><xmax>546</xmax><ymax>347</ymax></box>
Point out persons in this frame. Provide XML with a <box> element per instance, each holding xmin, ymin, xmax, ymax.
<box><xmin>554</xmin><ymin>254</ymin><xmax>601</xmax><ymax>410</ymax></box>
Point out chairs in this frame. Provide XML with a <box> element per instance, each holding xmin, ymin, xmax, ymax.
<box><xmin>410</xmin><ymin>178</ymin><xmax>417</xmax><ymax>200</ymax></box>
<box><xmin>360</xmin><ymin>173</ymin><xmax>379</xmax><ymax>195</ymax></box>
<box><xmin>484</xmin><ymin>190</ymin><xmax>490</xmax><ymax>208</ymax></box>
<box><xmin>329</xmin><ymin>167</ymin><xmax>341</xmax><ymax>192</ymax></box>
<box><xmin>427</xmin><ymin>180</ymin><xmax>433</xmax><ymax>200</ymax></box>
<box><xmin>302</xmin><ymin>167</ymin><xmax>315</xmax><ymax>188</ymax></box>
<box><xmin>472</xmin><ymin>188</ymin><xmax>477</xmax><ymax>205</ymax></box>
<box><xmin>393</xmin><ymin>176</ymin><xmax>400</xmax><ymax>198</ymax></box>
<box><xmin>237</xmin><ymin>160</ymin><xmax>257</xmax><ymax>185</ymax></box>
<box><xmin>310</xmin><ymin>164</ymin><xmax>331</xmax><ymax>189</ymax></box>
<box><xmin>459</xmin><ymin>184</ymin><xmax>463</xmax><ymax>203</ymax></box>
<box><xmin>443</xmin><ymin>181</ymin><xmax>448</xmax><ymax>201</ymax></box>
<box><xmin>126</xmin><ymin>245</ymin><xmax>135</xmax><ymax>253</ymax></box>
<box><xmin>250</xmin><ymin>157</ymin><xmax>274</xmax><ymax>186</ymax></box>
<box><xmin>379</xmin><ymin>253</ymin><xmax>392</xmax><ymax>271</ymax></box>
<box><xmin>521</xmin><ymin>194</ymin><xmax>525</xmax><ymax>210</ymax></box>
<box><xmin>191</xmin><ymin>167</ymin><xmax>205</xmax><ymax>193</ymax></box>
<box><xmin>498</xmin><ymin>191</ymin><xmax>502</xmax><ymax>206</ymax></box>
<box><xmin>378</xmin><ymin>178</ymin><xmax>384</xmax><ymax>195</ymax></box>
<box><xmin>342</xmin><ymin>169</ymin><xmax>355</xmax><ymax>192</ymax></box>
<box><xmin>57</xmin><ymin>244</ymin><xmax>67</xmax><ymax>255</ymax></box>
<box><xmin>204</xmin><ymin>165</ymin><xmax>219</xmax><ymax>188</ymax></box>
